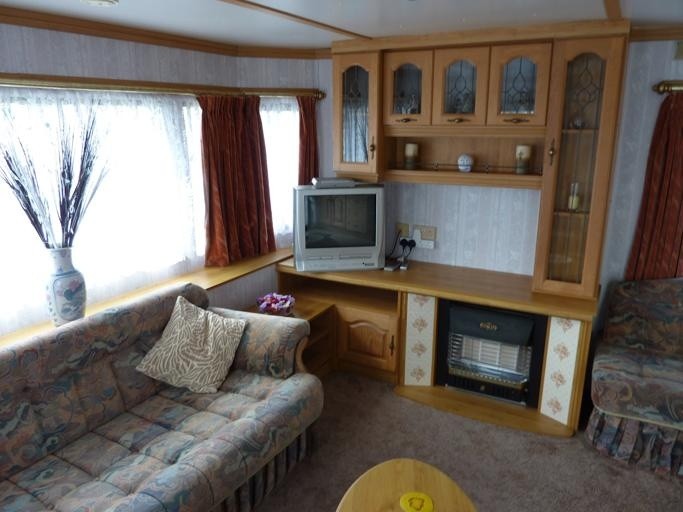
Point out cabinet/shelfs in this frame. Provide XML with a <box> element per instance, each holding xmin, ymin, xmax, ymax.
<box><xmin>332</xmin><ymin>49</ymin><xmax>383</xmax><ymax>178</ymax></box>
<box><xmin>337</xmin><ymin>309</ymin><xmax>400</xmax><ymax>367</ymax></box>
<box><xmin>535</xmin><ymin>33</ymin><xmax>627</xmax><ymax>297</ymax></box>
<box><xmin>385</xmin><ymin>40</ymin><xmax>548</xmax><ymax>187</ymax></box>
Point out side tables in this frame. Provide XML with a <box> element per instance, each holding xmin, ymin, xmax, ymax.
<box><xmin>243</xmin><ymin>295</ymin><xmax>336</xmax><ymax>377</ymax></box>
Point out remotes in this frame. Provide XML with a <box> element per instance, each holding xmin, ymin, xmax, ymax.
<box><xmin>384</xmin><ymin>263</ymin><xmax>400</xmax><ymax>270</ymax></box>
<box><xmin>400</xmin><ymin>263</ymin><xmax>407</xmax><ymax>271</ymax></box>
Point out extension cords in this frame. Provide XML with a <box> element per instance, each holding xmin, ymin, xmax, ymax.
<box><xmin>400</xmin><ymin>231</ymin><xmax>434</xmax><ymax>250</ymax></box>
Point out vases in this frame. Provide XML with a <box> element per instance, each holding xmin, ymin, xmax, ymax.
<box><xmin>46</xmin><ymin>247</ymin><xmax>84</xmax><ymax>327</ymax></box>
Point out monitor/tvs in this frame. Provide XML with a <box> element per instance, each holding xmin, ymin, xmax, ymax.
<box><xmin>294</xmin><ymin>184</ymin><xmax>386</xmax><ymax>272</ymax></box>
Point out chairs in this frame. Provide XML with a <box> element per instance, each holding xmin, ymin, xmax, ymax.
<box><xmin>591</xmin><ymin>280</ymin><xmax>683</xmax><ymax>470</ymax></box>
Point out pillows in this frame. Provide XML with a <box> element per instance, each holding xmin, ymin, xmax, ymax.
<box><xmin>133</xmin><ymin>295</ymin><xmax>249</xmax><ymax>397</ymax></box>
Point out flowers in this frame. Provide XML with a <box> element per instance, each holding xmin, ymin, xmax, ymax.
<box><xmin>257</xmin><ymin>289</ymin><xmax>295</xmax><ymax>312</ymax></box>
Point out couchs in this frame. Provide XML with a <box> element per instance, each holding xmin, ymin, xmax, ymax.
<box><xmin>0</xmin><ymin>282</ymin><xmax>327</xmax><ymax>510</ymax></box>
